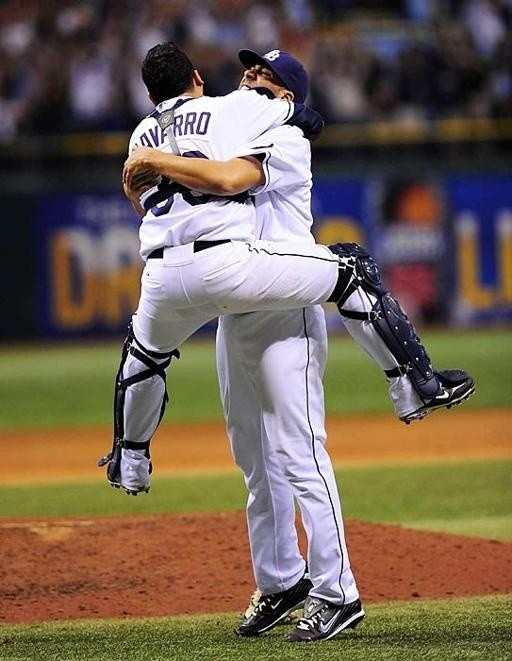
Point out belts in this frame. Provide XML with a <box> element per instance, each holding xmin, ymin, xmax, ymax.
<box><xmin>148</xmin><ymin>238</ymin><xmax>229</xmax><ymax>259</ymax></box>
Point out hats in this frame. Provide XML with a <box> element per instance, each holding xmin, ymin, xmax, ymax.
<box><xmin>238</xmin><ymin>48</ymin><xmax>309</xmax><ymax>104</ymax></box>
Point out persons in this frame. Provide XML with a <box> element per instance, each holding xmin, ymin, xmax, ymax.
<box><xmin>118</xmin><ymin>47</ymin><xmax>365</xmax><ymax>645</ymax></box>
<box><xmin>97</xmin><ymin>41</ymin><xmax>476</xmax><ymax>499</ymax></box>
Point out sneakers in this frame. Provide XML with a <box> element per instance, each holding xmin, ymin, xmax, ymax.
<box><xmin>399</xmin><ymin>377</ymin><xmax>477</xmax><ymax>426</ymax></box>
<box><xmin>107</xmin><ymin>461</ymin><xmax>150</xmax><ymax>496</ymax></box>
<box><xmin>232</xmin><ymin>562</ymin><xmax>313</xmax><ymax>637</ymax></box>
<box><xmin>284</xmin><ymin>595</ymin><xmax>365</xmax><ymax>642</ymax></box>
